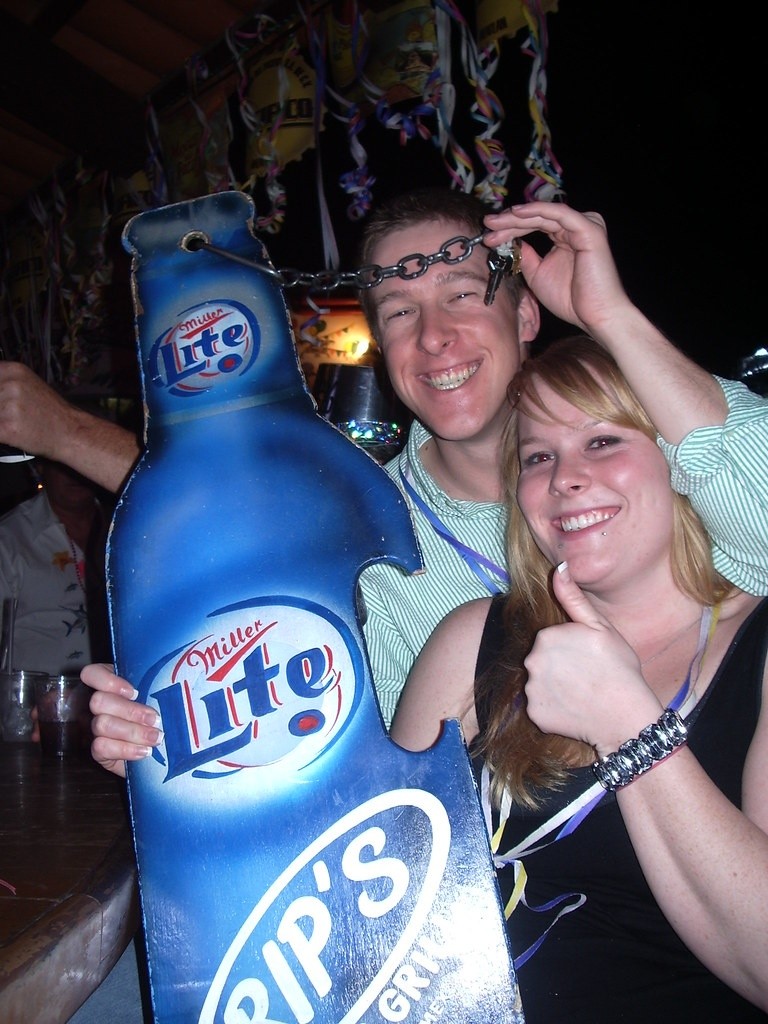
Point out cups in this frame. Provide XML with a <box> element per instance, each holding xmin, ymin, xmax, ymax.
<box><xmin>0</xmin><ymin>670</ymin><xmax>51</xmax><ymax>743</ymax></box>
<box><xmin>33</xmin><ymin>677</ymin><xmax>90</xmax><ymax>771</ymax></box>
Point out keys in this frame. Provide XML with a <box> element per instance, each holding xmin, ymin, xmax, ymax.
<box><xmin>483</xmin><ymin>245</ymin><xmax>516</xmax><ymax>307</ymax></box>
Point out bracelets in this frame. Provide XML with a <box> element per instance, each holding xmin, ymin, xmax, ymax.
<box><xmin>592</xmin><ymin>708</ymin><xmax>691</xmax><ymax>792</ymax></box>
<box><xmin>616</xmin><ymin>742</ymin><xmax>688</xmax><ymax>792</ymax></box>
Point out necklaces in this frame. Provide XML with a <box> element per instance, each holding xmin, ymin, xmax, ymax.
<box><xmin>64</xmin><ymin>529</ymin><xmax>87</xmax><ymax>598</ymax></box>
<box><xmin>477</xmin><ymin>602</ymin><xmax>722</xmax><ymax>970</ymax></box>
<box><xmin>397</xmin><ymin>448</ymin><xmax>514</xmax><ymax>599</ymax></box>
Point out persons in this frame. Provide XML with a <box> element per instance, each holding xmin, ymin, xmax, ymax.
<box><xmin>0</xmin><ymin>362</ymin><xmax>140</xmax><ymax>496</ymax></box>
<box><xmin>0</xmin><ymin>456</ymin><xmax>111</xmax><ymax>677</ymax></box>
<box><xmin>349</xmin><ymin>188</ymin><xmax>768</xmax><ymax>739</ymax></box>
<box><xmin>82</xmin><ymin>334</ymin><xmax>768</xmax><ymax>1024</ymax></box>
<box><xmin>29</xmin><ymin>685</ymin><xmax>97</xmax><ymax>743</ymax></box>
